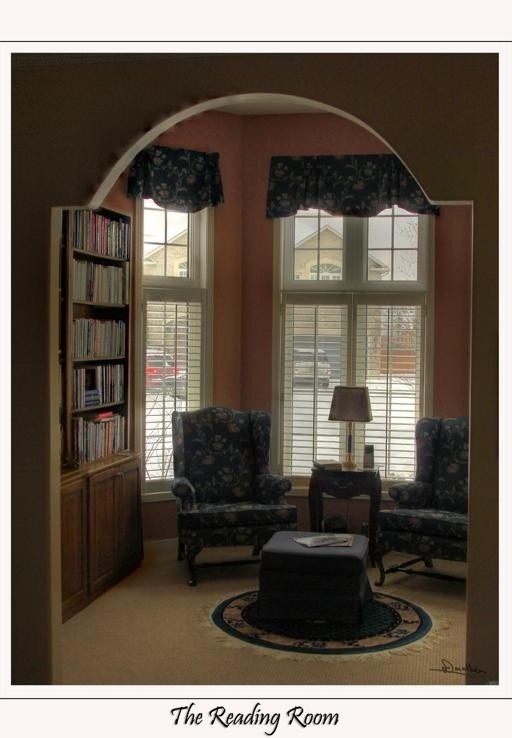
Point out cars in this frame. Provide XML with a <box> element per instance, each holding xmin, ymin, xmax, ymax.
<box><xmin>294</xmin><ymin>348</ymin><xmax>331</xmax><ymax>388</ymax></box>
<box><xmin>147</xmin><ymin>353</ymin><xmax>178</xmax><ymax>387</ymax></box>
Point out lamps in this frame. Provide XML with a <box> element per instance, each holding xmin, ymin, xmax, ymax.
<box><xmin>328</xmin><ymin>387</ymin><xmax>373</xmax><ymax>468</ymax></box>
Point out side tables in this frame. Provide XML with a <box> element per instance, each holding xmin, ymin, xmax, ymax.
<box><xmin>309</xmin><ymin>466</ymin><xmax>381</xmax><ymax>568</ymax></box>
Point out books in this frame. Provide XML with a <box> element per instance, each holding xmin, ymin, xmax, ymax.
<box><xmin>292</xmin><ymin>534</ymin><xmax>347</xmax><ymax>549</ymax></box>
<box><xmin>328</xmin><ymin>535</ymin><xmax>353</xmax><ymax>547</ymax></box>
<box><xmin>312</xmin><ymin>459</ymin><xmax>341</xmax><ymax>471</ymax></box>
<box><xmin>66</xmin><ymin>209</ymin><xmax>131</xmax><ymax>466</ymax></box>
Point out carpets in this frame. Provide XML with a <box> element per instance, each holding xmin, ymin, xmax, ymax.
<box><xmin>190</xmin><ymin>586</ymin><xmax>454</xmax><ymax>664</ymax></box>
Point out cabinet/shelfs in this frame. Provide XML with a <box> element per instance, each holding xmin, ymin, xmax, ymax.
<box><xmin>61</xmin><ymin>206</ymin><xmax>143</xmax><ymax>627</ymax></box>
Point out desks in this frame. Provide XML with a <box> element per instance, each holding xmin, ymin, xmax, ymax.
<box><xmin>259</xmin><ymin>531</ymin><xmax>370</xmax><ymax>624</ymax></box>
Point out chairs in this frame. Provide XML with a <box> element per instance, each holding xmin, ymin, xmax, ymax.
<box><xmin>373</xmin><ymin>417</ymin><xmax>469</xmax><ymax>587</ymax></box>
<box><xmin>172</xmin><ymin>406</ymin><xmax>298</xmax><ymax>588</ymax></box>
